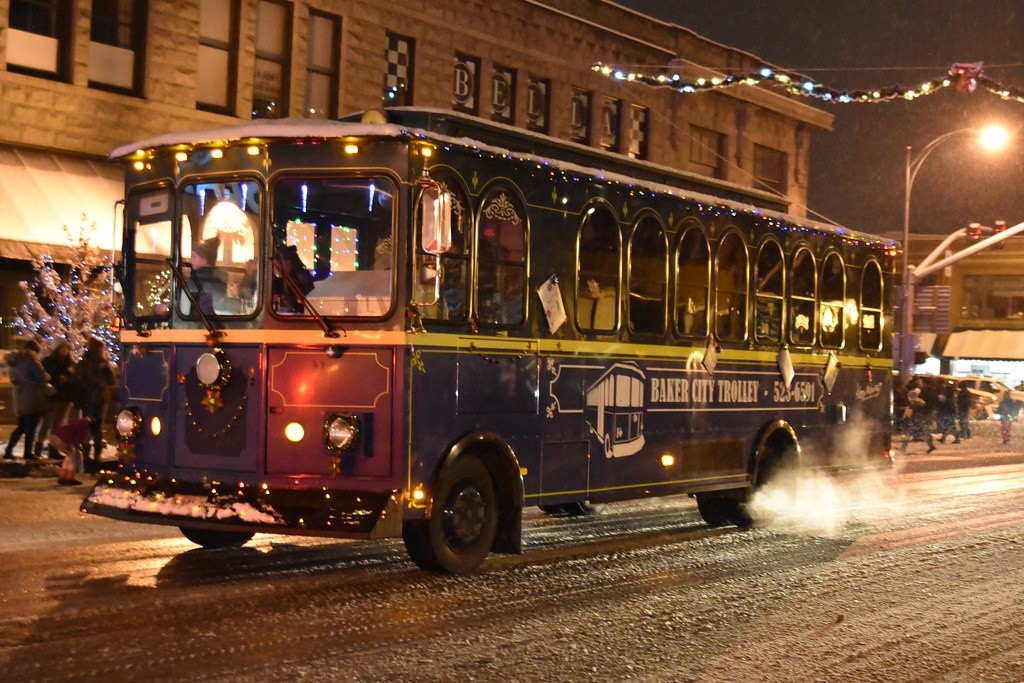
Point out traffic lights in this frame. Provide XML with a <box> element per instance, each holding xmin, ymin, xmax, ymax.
<box><xmin>992</xmin><ymin>219</ymin><xmax>1007</xmax><ymax>249</ymax></box>
<box><xmin>966</xmin><ymin>218</ymin><xmax>982</xmax><ymax>246</ymax></box>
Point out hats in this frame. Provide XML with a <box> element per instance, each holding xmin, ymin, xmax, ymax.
<box><xmin>908</xmin><ymin>388</ymin><xmax>921</xmax><ymax>398</ymax></box>
<box><xmin>53</xmin><ymin>337</ymin><xmax>69</xmax><ymax>349</ymax></box>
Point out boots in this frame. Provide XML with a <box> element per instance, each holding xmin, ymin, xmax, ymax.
<box><xmin>47</xmin><ymin>443</ymin><xmax>67</xmax><ymax>458</ymax></box>
<box><xmin>35</xmin><ymin>442</ymin><xmax>49</xmax><ymax>455</ymax></box>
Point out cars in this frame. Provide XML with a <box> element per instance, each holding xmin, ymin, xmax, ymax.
<box><xmin>928</xmin><ymin>375</ymin><xmax>1024</xmax><ymax>421</ymax></box>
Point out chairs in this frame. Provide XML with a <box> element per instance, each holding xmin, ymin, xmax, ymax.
<box><xmin>561</xmin><ymin>286</ymin><xmax>842</xmax><ymax>346</ymax></box>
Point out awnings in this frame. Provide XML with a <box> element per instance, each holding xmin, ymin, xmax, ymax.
<box><xmin>891</xmin><ymin>333</ymin><xmax>938</xmax><ymax>356</ymax></box>
<box><xmin>0</xmin><ymin>144</ymin><xmax>356</xmax><ymax>281</ymax></box>
<box><xmin>942</xmin><ymin>330</ymin><xmax>1024</xmax><ymax>359</ymax></box>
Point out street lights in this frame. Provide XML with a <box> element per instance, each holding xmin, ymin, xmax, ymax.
<box><xmin>905</xmin><ymin>123</ymin><xmax>1012</xmax><ymax>445</ymax></box>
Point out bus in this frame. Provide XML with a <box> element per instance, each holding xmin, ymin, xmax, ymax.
<box><xmin>81</xmin><ymin>104</ymin><xmax>903</xmax><ymax>575</ymax></box>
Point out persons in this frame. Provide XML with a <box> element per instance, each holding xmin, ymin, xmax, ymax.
<box><xmin>3</xmin><ymin>339</ymin><xmax>50</xmax><ymax>460</ymax></box>
<box><xmin>998</xmin><ymin>391</ymin><xmax>1018</xmax><ymax>445</ymax></box>
<box><xmin>239</xmin><ymin>227</ymin><xmax>316</xmax><ymax>314</ymax></box>
<box><xmin>75</xmin><ymin>334</ymin><xmax>116</xmax><ymax>464</ymax></box>
<box><xmin>33</xmin><ymin>339</ymin><xmax>76</xmax><ymax>460</ymax></box>
<box><xmin>187</xmin><ymin>238</ymin><xmax>229</xmax><ymax>315</ymax></box>
<box><xmin>437</xmin><ymin>226</ymin><xmax>488</xmax><ymax>321</ymax></box>
<box><xmin>894</xmin><ymin>379</ymin><xmax>971</xmax><ymax>453</ymax></box>
<box><xmin>50</xmin><ymin>417</ymin><xmax>98</xmax><ymax>486</ymax></box>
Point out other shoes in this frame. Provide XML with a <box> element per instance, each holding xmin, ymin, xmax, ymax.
<box><xmin>936</xmin><ymin>437</ymin><xmax>944</xmax><ymax>443</ymax></box>
<box><xmin>951</xmin><ymin>438</ymin><xmax>960</xmax><ymax>444</ymax></box>
<box><xmin>23</xmin><ymin>452</ymin><xmax>38</xmax><ymax>458</ymax></box>
<box><xmin>926</xmin><ymin>446</ymin><xmax>936</xmax><ymax>453</ymax></box>
<box><xmin>3</xmin><ymin>453</ymin><xmax>17</xmax><ymax>459</ymax></box>
